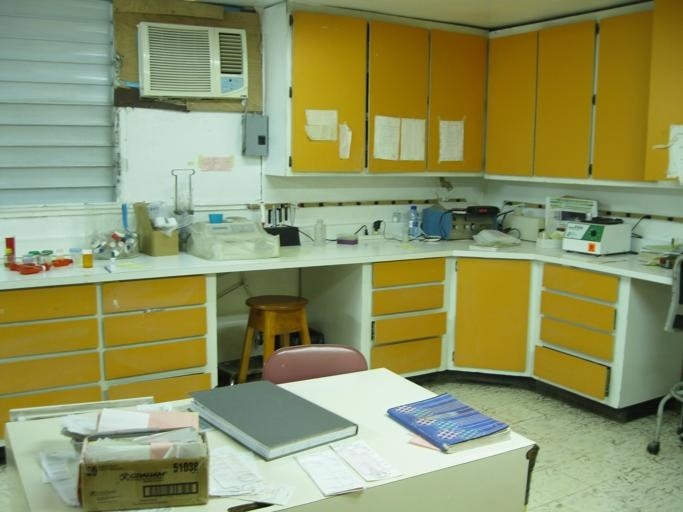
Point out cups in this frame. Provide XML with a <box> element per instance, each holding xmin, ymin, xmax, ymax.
<box><xmin>207</xmin><ymin>213</ymin><xmax>223</xmax><ymax>223</ymax></box>
<box><xmin>21</xmin><ymin>249</ymin><xmax>54</xmax><ymax>269</ymax></box>
<box><xmin>171</xmin><ymin>168</ymin><xmax>195</xmax><ymax>253</ymax></box>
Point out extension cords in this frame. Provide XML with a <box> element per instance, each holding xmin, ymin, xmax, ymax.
<box><xmin>359</xmin><ymin>232</ymin><xmax>386</xmax><ymax>239</ymax></box>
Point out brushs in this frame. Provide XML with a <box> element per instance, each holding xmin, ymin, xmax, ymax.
<box><xmin>336</xmin><ymin>233</ymin><xmax>358</xmax><ymax>244</ymax></box>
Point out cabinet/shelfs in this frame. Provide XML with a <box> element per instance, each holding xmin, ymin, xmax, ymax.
<box><xmin>426</xmin><ymin>21</ymin><xmax>486</xmax><ymax>176</ymax></box>
<box><xmin>586</xmin><ymin>1</ymin><xmax>682</xmax><ymax>189</ymax></box>
<box><xmin>92</xmin><ymin>247</ymin><xmax>219</xmax><ymax>412</ymax></box>
<box><xmin>0</xmin><ymin>260</ymin><xmax>104</xmax><ymax>468</ymax></box>
<box><xmin>450</xmin><ymin>237</ymin><xmax>535</xmax><ymax>394</ymax></box>
<box><xmin>297</xmin><ymin>238</ymin><xmax>450</xmax><ymax>387</ymax></box>
<box><xmin>255</xmin><ymin>2</ymin><xmax>369</xmax><ymax>176</ymax></box>
<box><xmin>529</xmin><ymin>243</ymin><xmax>679</xmax><ymax>424</ymax></box>
<box><xmin>364</xmin><ymin>8</ymin><xmax>428</xmax><ymax>178</ymax></box>
<box><xmin>483</xmin><ymin>11</ymin><xmax>596</xmax><ymax>183</ymax></box>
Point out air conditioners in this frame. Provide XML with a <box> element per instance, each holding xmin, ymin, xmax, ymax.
<box><xmin>132</xmin><ymin>19</ymin><xmax>249</xmax><ymax>108</ymax></box>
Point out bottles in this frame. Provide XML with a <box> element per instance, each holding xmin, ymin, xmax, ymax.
<box><xmin>314</xmin><ymin>218</ymin><xmax>329</xmax><ymax>247</ymax></box>
<box><xmin>79</xmin><ymin>249</ymin><xmax>93</xmax><ymax>269</ymax></box>
<box><xmin>2</xmin><ymin>248</ymin><xmax>14</xmax><ymax>267</ymax></box>
<box><xmin>406</xmin><ymin>205</ymin><xmax>422</xmax><ymax>243</ymax></box>
<box><xmin>537</xmin><ymin>228</ymin><xmax>549</xmax><ymax>239</ymax></box>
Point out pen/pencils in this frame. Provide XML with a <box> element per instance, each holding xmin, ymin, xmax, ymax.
<box><xmin>104</xmin><ymin>266</ymin><xmax>111</xmax><ymax>273</ymax></box>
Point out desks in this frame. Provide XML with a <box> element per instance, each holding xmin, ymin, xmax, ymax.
<box><xmin>2</xmin><ymin>365</ymin><xmax>540</xmax><ymax>511</ymax></box>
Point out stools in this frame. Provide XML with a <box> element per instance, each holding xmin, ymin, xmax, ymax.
<box><xmin>230</xmin><ymin>295</ymin><xmax>310</xmax><ymax>385</ymax></box>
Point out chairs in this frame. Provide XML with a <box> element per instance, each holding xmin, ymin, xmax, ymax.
<box><xmin>259</xmin><ymin>342</ymin><xmax>368</xmax><ymax>387</ymax></box>
<box><xmin>641</xmin><ymin>253</ymin><xmax>683</xmax><ymax>454</ymax></box>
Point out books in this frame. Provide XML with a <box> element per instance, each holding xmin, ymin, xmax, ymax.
<box><xmin>95</xmin><ymin>403</ymin><xmax>200</xmax><ymax>435</ymax></box>
<box><xmin>387</xmin><ymin>392</ymin><xmax>513</xmax><ymax>457</ymax></box>
<box><xmin>187</xmin><ymin>380</ymin><xmax>358</xmax><ymax>461</ymax></box>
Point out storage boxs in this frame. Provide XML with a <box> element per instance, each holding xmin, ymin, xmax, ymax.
<box><xmin>72</xmin><ymin>425</ymin><xmax>211</xmax><ymax>512</ymax></box>
<box><xmin>131</xmin><ymin>200</ymin><xmax>179</xmax><ymax>258</ymax></box>
